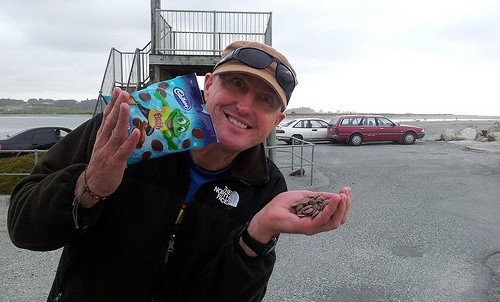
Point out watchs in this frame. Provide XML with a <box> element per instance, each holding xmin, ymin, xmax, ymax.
<box><xmin>242</xmin><ymin>222</ymin><xmax>278</xmax><ymax>256</ymax></box>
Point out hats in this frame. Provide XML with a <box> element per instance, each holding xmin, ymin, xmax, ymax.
<box><xmin>212</xmin><ymin>40</ymin><xmax>299</xmax><ymax>112</ymax></box>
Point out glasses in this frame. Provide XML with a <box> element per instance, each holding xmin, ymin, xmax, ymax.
<box><xmin>213</xmin><ymin>46</ymin><xmax>299</xmax><ymax>94</ymax></box>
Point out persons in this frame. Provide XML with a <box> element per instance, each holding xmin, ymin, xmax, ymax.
<box><xmin>6</xmin><ymin>40</ymin><xmax>350</xmax><ymax>302</ymax></box>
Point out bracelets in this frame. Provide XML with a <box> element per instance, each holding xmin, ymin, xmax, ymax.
<box><xmin>72</xmin><ymin>167</ymin><xmax>108</xmax><ymax>229</ymax></box>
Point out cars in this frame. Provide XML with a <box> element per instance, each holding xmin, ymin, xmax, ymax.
<box><xmin>0</xmin><ymin>126</ymin><xmax>72</xmax><ymax>159</ymax></box>
<box><xmin>327</xmin><ymin>115</ymin><xmax>425</xmax><ymax>146</ymax></box>
<box><xmin>275</xmin><ymin>118</ymin><xmax>329</xmax><ymax>145</ymax></box>
